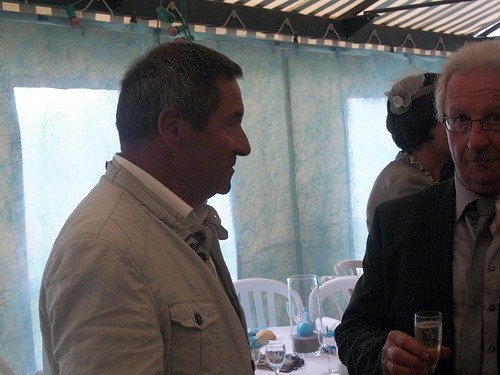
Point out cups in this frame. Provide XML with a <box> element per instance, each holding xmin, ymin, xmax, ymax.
<box><xmin>286</xmin><ymin>274</ymin><xmax>326</xmax><ymax>356</ymax></box>
<box><xmin>414</xmin><ymin>310</ymin><xmax>443</xmax><ymax>375</ymax></box>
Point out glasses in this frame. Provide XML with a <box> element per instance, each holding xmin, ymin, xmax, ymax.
<box><xmin>443</xmin><ymin>112</ymin><xmax>500</xmax><ymax>133</ymax></box>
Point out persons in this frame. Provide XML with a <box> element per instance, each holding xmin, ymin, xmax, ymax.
<box><xmin>334</xmin><ymin>40</ymin><xmax>500</xmax><ymax>375</ymax></box>
<box><xmin>366</xmin><ymin>73</ymin><xmax>451</xmax><ymax>233</ymax></box>
<box><xmin>39</xmin><ymin>42</ymin><xmax>257</xmax><ymax>375</ymax></box>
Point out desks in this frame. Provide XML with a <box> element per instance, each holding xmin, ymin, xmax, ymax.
<box><xmin>254</xmin><ymin>326</ymin><xmax>349</xmax><ymax>375</ymax></box>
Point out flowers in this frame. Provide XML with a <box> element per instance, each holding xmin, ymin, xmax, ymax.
<box><xmin>385</xmin><ymin>84</ymin><xmax>411</xmax><ymax>108</ymax></box>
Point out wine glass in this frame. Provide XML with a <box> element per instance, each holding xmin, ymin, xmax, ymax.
<box><xmin>251</xmin><ymin>349</ymin><xmax>260</xmax><ymax>375</ymax></box>
<box><xmin>316</xmin><ymin>329</ymin><xmax>341</xmax><ymax>375</ymax></box>
<box><xmin>265</xmin><ymin>343</ymin><xmax>286</xmax><ymax>375</ymax></box>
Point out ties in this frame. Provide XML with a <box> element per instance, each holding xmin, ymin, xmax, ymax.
<box><xmin>458</xmin><ymin>196</ymin><xmax>496</xmax><ymax>375</ymax></box>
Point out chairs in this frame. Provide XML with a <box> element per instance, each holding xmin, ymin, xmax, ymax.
<box><xmin>233</xmin><ymin>278</ymin><xmax>304</xmax><ymax>330</ymax></box>
<box><xmin>309</xmin><ymin>260</ymin><xmax>365</xmax><ymax>322</ymax></box>
